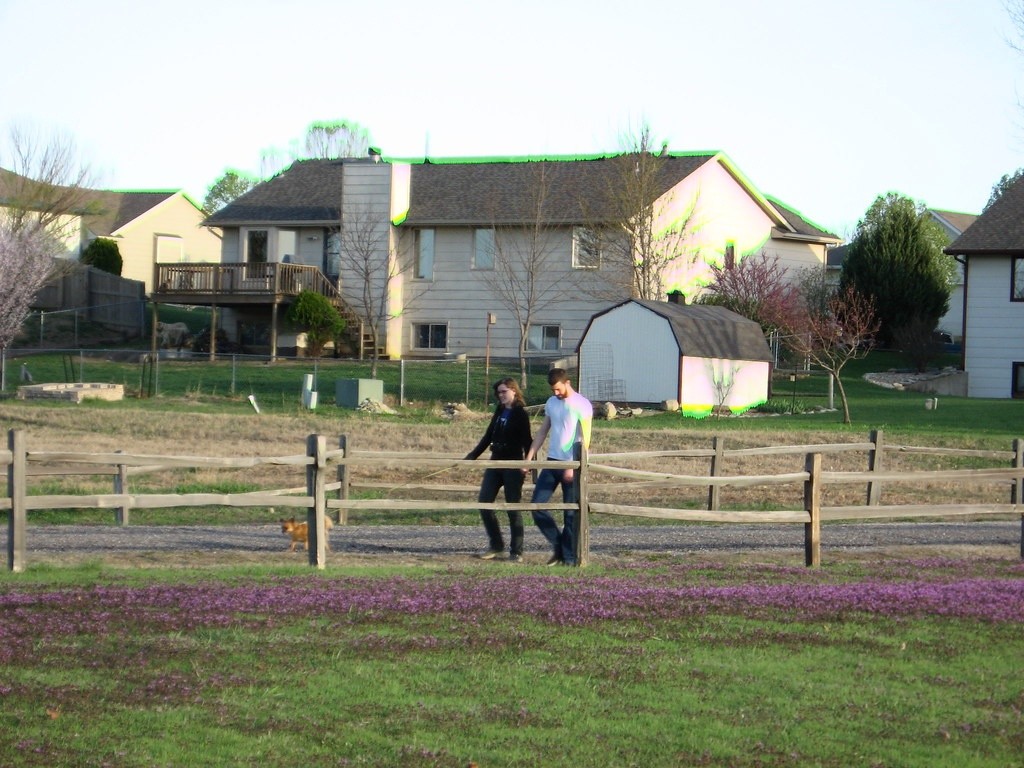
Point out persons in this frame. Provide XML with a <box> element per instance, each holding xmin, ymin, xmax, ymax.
<box><xmin>453</xmin><ymin>377</ymin><xmax>533</xmax><ymax>562</ymax></box>
<box><xmin>520</xmin><ymin>368</ymin><xmax>592</xmax><ymax>567</ymax></box>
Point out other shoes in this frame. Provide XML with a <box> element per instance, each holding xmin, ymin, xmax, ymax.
<box><xmin>509</xmin><ymin>555</ymin><xmax>522</xmax><ymax>563</ymax></box>
<box><xmin>546</xmin><ymin>556</ymin><xmax>564</xmax><ymax>567</ymax></box>
<box><xmin>480</xmin><ymin>548</ymin><xmax>504</xmax><ymax>559</ymax></box>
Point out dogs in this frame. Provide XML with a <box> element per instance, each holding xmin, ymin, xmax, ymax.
<box><xmin>279</xmin><ymin>515</ymin><xmax>334</xmax><ymax>553</ymax></box>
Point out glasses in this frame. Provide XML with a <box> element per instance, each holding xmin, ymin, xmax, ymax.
<box><xmin>497</xmin><ymin>389</ymin><xmax>511</xmax><ymax>395</ymax></box>
